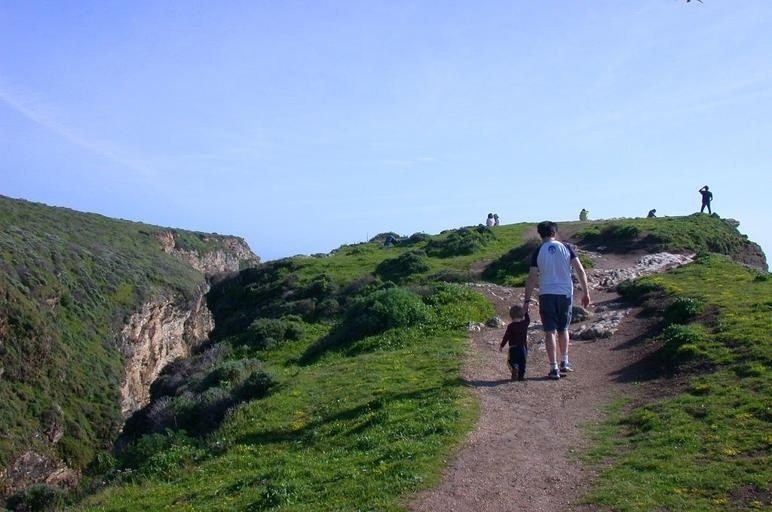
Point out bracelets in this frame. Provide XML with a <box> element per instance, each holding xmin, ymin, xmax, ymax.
<box><xmin>524</xmin><ymin>299</ymin><xmax>532</xmax><ymax>304</ymax></box>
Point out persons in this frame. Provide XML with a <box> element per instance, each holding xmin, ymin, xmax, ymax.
<box><xmin>493</xmin><ymin>213</ymin><xmax>502</xmax><ymax>225</ymax></box>
<box><xmin>522</xmin><ymin>220</ymin><xmax>591</xmax><ymax>379</ymax></box>
<box><xmin>486</xmin><ymin>213</ymin><xmax>496</xmax><ymax>226</ymax></box>
<box><xmin>499</xmin><ymin>304</ymin><xmax>531</xmax><ymax>381</ymax></box>
<box><xmin>579</xmin><ymin>208</ymin><xmax>591</xmax><ymax>221</ymax></box>
<box><xmin>647</xmin><ymin>208</ymin><xmax>657</xmax><ymax>218</ymax></box>
<box><xmin>698</xmin><ymin>184</ymin><xmax>713</xmax><ymax>214</ymax></box>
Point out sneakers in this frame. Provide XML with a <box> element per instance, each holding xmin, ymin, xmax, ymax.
<box><xmin>559</xmin><ymin>360</ymin><xmax>575</xmax><ymax>373</ymax></box>
<box><xmin>547</xmin><ymin>369</ymin><xmax>561</xmax><ymax>379</ymax></box>
<box><xmin>511</xmin><ymin>366</ymin><xmax>526</xmax><ymax>381</ymax></box>
